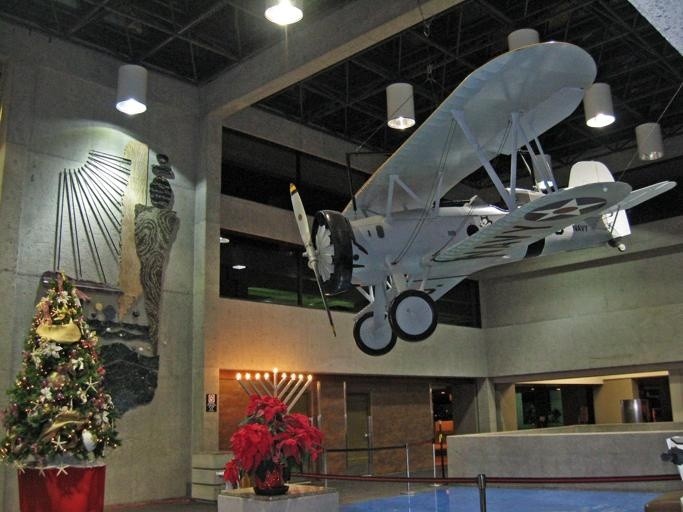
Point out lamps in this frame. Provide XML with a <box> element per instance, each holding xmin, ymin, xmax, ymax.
<box><xmin>113</xmin><ymin>32</ymin><xmax>149</xmax><ymax>118</ymax></box>
<box><xmin>384</xmin><ymin>30</ymin><xmax>416</xmax><ymax>131</ymax></box>
<box><xmin>583</xmin><ymin>82</ymin><xmax>615</xmax><ymax>129</ymax></box>
<box><xmin>507</xmin><ymin>21</ymin><xmax>539</xmax><ymax>52</ymax></box>
<box><xmin>632</xmin><ymin>105</ymin><xmax>665</xmax><ymax>162</ymax></box>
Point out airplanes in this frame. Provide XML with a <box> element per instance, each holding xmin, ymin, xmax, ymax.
<box><xmin>289</xmin><ymin>41</ymin><xmax>678</xmax><ymax>356</ymax></box>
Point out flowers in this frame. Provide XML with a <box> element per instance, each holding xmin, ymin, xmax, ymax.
<box><xmin>221</xmin><ymin>392</ymin><xmax>325</xmax><ymax>483</ymax></box>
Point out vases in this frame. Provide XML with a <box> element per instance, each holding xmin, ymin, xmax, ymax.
<box><xmin>244</xmin><ymin>460</ymin><xmax>291</xmax><ymax>495</ymax></box>
